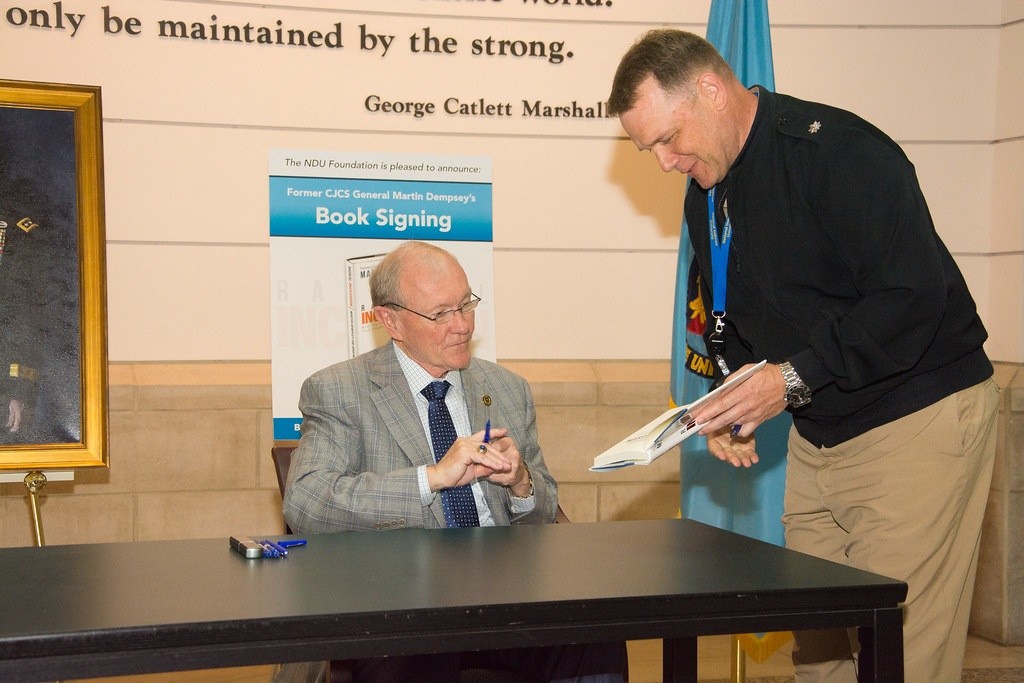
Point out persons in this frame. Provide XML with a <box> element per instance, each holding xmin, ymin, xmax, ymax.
<box><xmin>282</xmin><ymin>241</ymin><xmax>629</xmax><ymax>683</ymax></box>
<box><xmin>605</xmin><ymin>30</ymin><xmax>1001</xmax><ymax>683</ymax></box>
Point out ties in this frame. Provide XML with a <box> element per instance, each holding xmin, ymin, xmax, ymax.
<box><xmin>420</xmin><ymin>381</ymin><xmax>481</xmax><ymax>528</ymax></box>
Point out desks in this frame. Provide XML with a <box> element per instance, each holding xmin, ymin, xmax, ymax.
<box><xmin>0</xmin><ymin>518</ymin><xmax>909</xmax><ymax>683</ymax></box>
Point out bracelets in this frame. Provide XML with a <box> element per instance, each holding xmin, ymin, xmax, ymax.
<box><xmin>509</xmin><ymin>466</ymin><xmax>534</xmax><ymax>499</ymax></box>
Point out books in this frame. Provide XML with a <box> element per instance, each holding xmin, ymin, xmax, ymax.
<box><xmin>588</xmin><ymin>359</ymin><xmax>768</xmax><ymax>473</ymax></box>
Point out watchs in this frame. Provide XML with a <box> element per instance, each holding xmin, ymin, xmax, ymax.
<box><xmin>779</xmin><ymin>361</ymin><xmax>811</xmax><ymax>408</ymax></box>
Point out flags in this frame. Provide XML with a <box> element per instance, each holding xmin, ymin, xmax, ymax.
<box><xmin>667</xmin><ymin>0</ymin><xmax>793</xmax><ymax>662</ymax></box>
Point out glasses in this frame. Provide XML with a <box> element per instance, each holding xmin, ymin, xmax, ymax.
<box><xmin>380</xmin><ymin>293</ymin><xmax>481</xmax><ymax>325</ymax></box>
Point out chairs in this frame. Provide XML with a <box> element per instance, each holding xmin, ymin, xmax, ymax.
<box><xmin>270</xmin><ymin>444</ymin><xmax>572</xmax><ymax>535</ymax></box>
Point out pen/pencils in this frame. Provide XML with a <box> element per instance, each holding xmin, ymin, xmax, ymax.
<box><xmin>256</xmin><ymin>538</ymin><xmax>307</xmax><ymax>558</ymax></box>
<box><xmin>484</xmin><ymin>418</ymin><xmax>492</xmax><ymax>444</ymax></box>
<box><xmin>729</xmin><ymin>424</ymin><xmax>741</xmax><ymax>440</ymax></box>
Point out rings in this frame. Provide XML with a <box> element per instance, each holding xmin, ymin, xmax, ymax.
<box><xmin>478</xmin><ymin>445</ymin><xmax>487</xmax><ymax>454</ymax></box>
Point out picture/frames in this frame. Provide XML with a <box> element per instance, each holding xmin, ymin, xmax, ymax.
<box><xmin>0</xmin><ymin>79</ymin><xmax>113</xmax><ymax>471</ymax></box>
